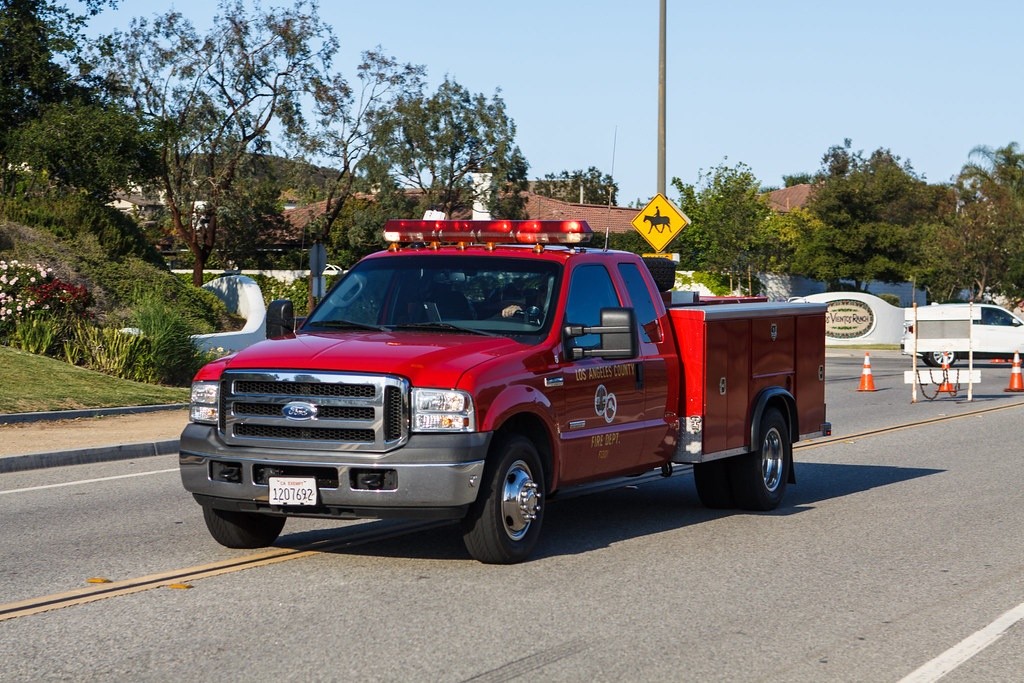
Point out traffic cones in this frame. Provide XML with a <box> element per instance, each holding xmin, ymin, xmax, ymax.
<box><xmin>854</xmin><ymin>352</ymin><xmax>880</xmax><ymax>391</ymax></box>
<box><xmin>935</xmin><ymin>352</ymin><xmax>957</xmax><ymax>392</ymax></box>
<box><xmin>1003</xmin><ymin>350</ymin><xmax>1024</xmax><ymax>393</ymax></box>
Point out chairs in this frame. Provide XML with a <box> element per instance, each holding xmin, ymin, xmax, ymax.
<box><xmin>411</xmin><ymin>280</ymin><xmax>477</xmax><ymax>322</ymax></box>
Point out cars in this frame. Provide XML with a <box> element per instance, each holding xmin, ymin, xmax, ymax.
<box><xmin>899</xmin><ymin>302</ymin><xmax>1024</xmax><ymax>367</ymax></box>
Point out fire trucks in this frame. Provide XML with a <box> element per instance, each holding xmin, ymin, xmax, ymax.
<box><xmin>179</xmin><ymin>215</ymin><xmax>836</xmax><ymax>566</ymax></box>
